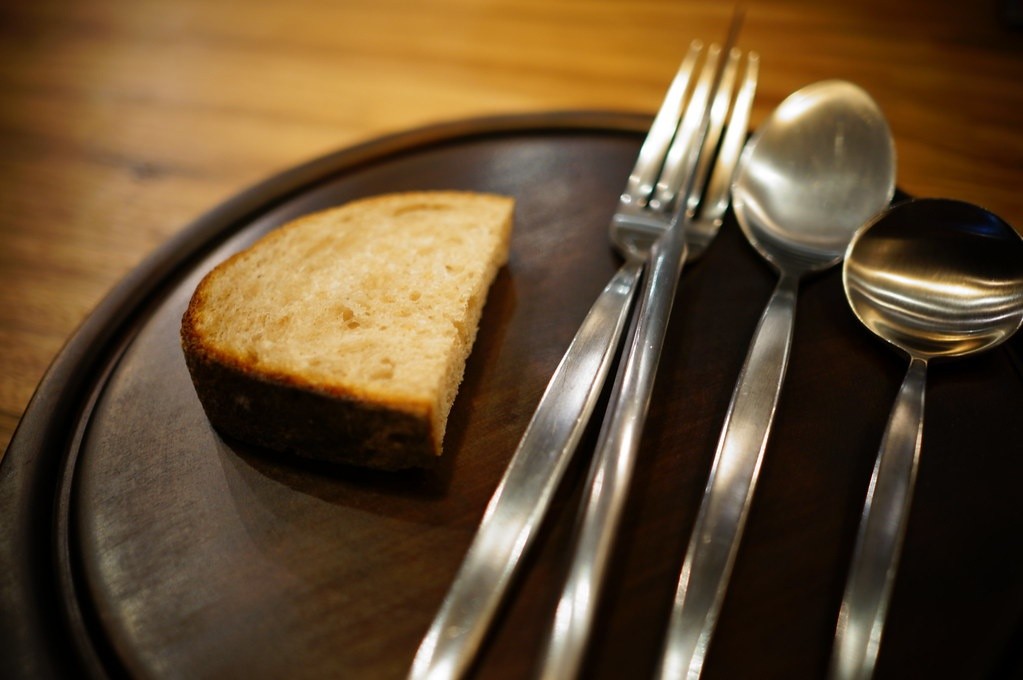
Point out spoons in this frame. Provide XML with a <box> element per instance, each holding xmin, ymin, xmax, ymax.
<box><xmin>831</xmin><ymin>193</ymin><xmax>1023</xmax><ymax>680</ymax></box>
<box><xmin>656</xmin><ymin>76</ymin><xmax>897</xmax><ymax>680</ymax></box>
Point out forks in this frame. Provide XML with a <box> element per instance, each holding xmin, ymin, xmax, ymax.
<box><xmin>405</xmin><ymin>38</ymin><xmax>761</xmax><ymax>680</ymax></box>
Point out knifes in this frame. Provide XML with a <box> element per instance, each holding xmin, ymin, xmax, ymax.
<box><xmin>541</xmin><ymin>0</ymin><xmax>753</xmax><ymax>680</ymax></box>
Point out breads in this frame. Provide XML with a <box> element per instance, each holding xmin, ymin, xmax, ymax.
<box><xmin>177</xmin><ymin>191</ymin><xmax>516</xmax><ymax>456</ymax></box>
